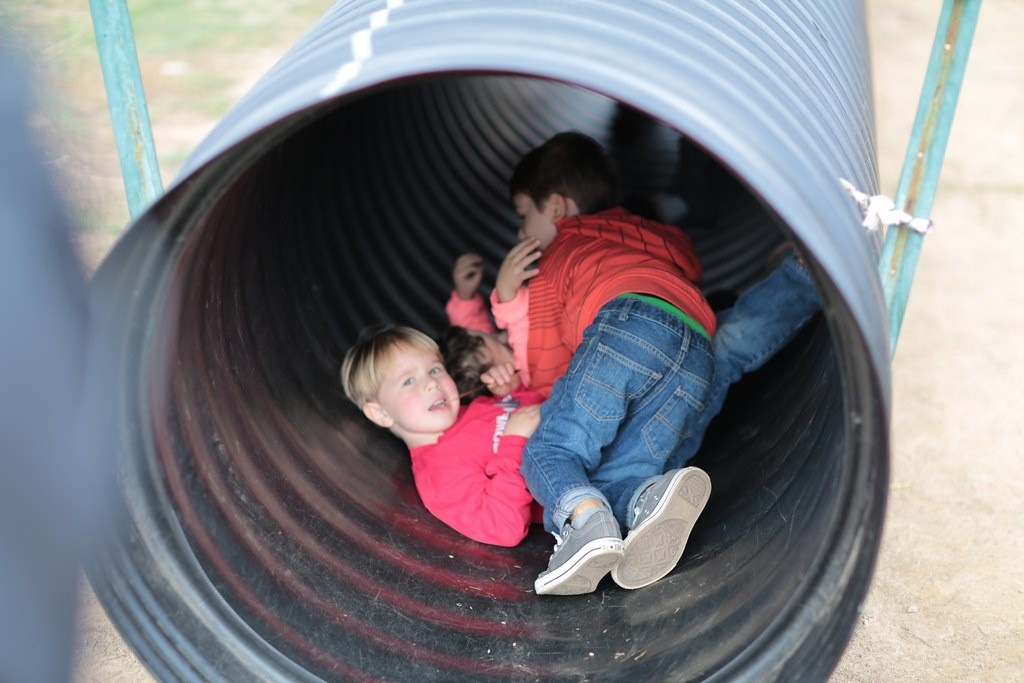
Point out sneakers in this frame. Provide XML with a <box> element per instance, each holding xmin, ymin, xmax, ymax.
<box><xmin>611</xmin><ymin>467</ymin><xmax>711</xmax><ymax>589</ymax></box>
<box><xmin>534</xmin><ymin>511</ymin><xmax>625</xmax><ymax>595</ymax></box>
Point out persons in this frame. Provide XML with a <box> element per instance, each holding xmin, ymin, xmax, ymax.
<box><xmin>339</xmin><ymin>235</ymin><xmax>825</xmax><ymax>549</ymax></box>
<box><xmin>509</xmin><ymin>130</ymin><xmax>717</xmax><ymax>596</ymax></box>
